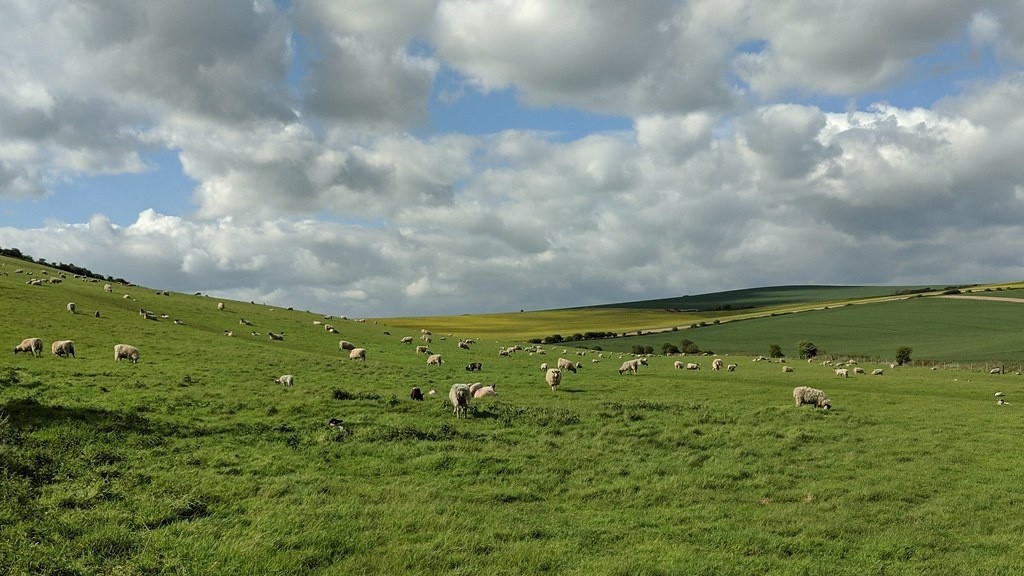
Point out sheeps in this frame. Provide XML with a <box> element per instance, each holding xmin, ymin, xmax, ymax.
<box><xmin>1</xmin><ymin>251</ymin><xmax>1012</xmax><ymax>420</ymax></box>
